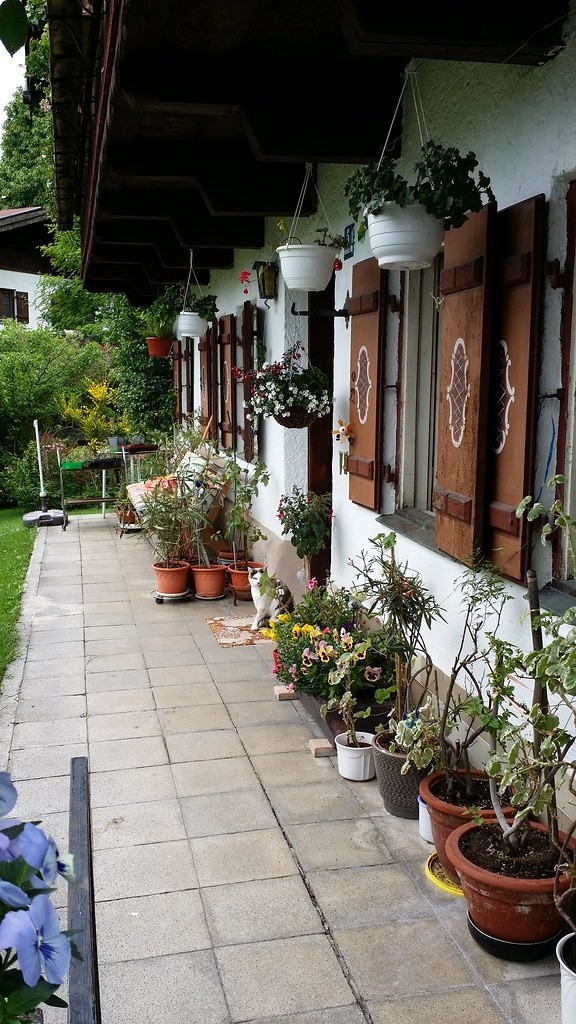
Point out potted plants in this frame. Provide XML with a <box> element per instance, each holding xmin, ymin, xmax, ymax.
<box><xmin>145</xmin><ymin>280</ymin><xmax>220</xmax><ymax>356</ymax></box>
<box><xmin>113</xmin><ymin>406</ymin><xmax>271</xmax><ymax>600</ymax></box>
<box><xmin>345</xmin><ymin>475</ymin><xmax>576</xmax><ymax>1024</ymax></box>
<box><xmin>343</xmin><ymin>140</ymin><xmax>497</xmax><ymax>271</ymax></box>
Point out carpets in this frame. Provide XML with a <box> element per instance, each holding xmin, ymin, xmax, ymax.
<box><xmin>205</xmin><ymin>614</ymin><xmax>277</xmax><ymax>649</ymax></box>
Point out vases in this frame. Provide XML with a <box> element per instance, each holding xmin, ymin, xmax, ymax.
<box><xmin>334</xmin><ymin>732</ymin><xmax>376</xmax><ymax>781</ymax></box>
<box><xmin>273</xmin><ymin>648</ymin><xmax>282</xmax><ymax>669</ymax></box>
<box><xmin>274</xmin><ymin>245</ymin><xmax>340</xmax><ymax>292</ymax></box>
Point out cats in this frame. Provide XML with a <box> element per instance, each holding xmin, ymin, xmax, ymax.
<box><xmin>246</xmin><ymin>565</ymin><xmax>294</xmax><ymax>631</ymax></box>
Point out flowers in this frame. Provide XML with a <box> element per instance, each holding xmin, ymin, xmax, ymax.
<box><xmin>255</xmin><ymin>570</ymin><xmax>416</xmax><ymax>746</ymax></box>
<box><xmin>239</xmin><ymin>219</ymin><xmax>354</xmax><ymax>294</ymax></box>
<box><xmin>0</xmin><ymin>772</ymin><xmax>85</xmax><ymax>1024</ymax></box>
<box><xmin>230</xmin><ymin>340</ymin><xmax>337</xmax><ymax>430</ymax></box>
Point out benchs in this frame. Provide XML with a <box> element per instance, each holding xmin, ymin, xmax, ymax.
<box><xmin>126</xmin><ymin>448</ymin><xmax>232</xmax><ymax>553</ymax></box>
